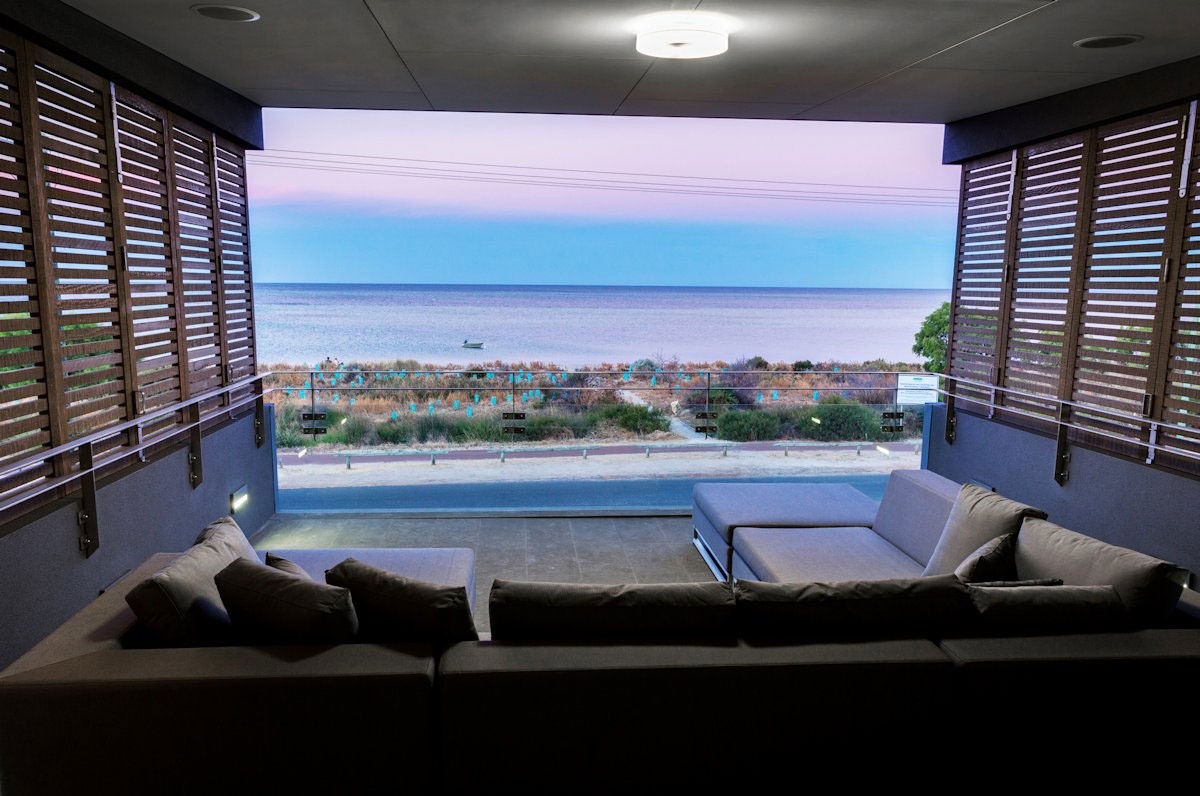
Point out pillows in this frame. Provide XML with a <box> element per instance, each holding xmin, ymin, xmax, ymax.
<box><xmin>953</xmin><ymin>533</ymin><xmax>1010</xmax><ymax>584</ymax></box>
<box><xmin>123</xmin><ymin>516</ymin><xmax>265</xmax><ymax>647</ymax></box>
<box><xmin>325</xmin><ymin>557</ymin><xmax>480</xmax><ymax>644</ymax></box>
<box><xmin>736</xmin><ymin>574</ymin><xmax>972</xmax><ymax>640</ymax></box>
<box><xmin>489</xmin><ymin>567</ymin><xmax>737</xmax><ymax>649</ymax></box>
<box><xmin>964</xmin><ymin>584</ymin><xmax>1141</xmax><ymax>638</ymax></box>
<box><xmin>919</xmin><ymin>482</ymin><xmax>1049</xmax><ymax>578</ymax></box>
<box><xmin>1010</xmin><ymin>515</ymin><xmax>1186</xmax><ymax>629</ymax></box>
<box><xmin>264</xmin><ymin>552</ymin><xmax>315</xmax><ymax>584</ymax></box>
<box><xmin>212</xmin><ymin>557</ymin><xmax>361</xmax><ymax>644</ymax></box>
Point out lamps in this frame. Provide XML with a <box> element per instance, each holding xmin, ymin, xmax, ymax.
<box><xmin>635</xmin><ymin>8</ymin><xmax>729</xmax><ymax>60</ymax></box>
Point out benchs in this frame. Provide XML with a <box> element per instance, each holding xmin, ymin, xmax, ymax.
<box><xmin>255</xmin><ymin>546</ymin><xmax>477</xmax><ymax>614</ymax></box>
<box><xmin>691</xmin><ymin>481</ymin><xmax>880</xmax><ymax>582</ymax></box>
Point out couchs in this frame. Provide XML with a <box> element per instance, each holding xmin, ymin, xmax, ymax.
<box><xmin>6</xmin><ymin>471</ymin><xmax>1199</xmax><ymax>796</ymax></box>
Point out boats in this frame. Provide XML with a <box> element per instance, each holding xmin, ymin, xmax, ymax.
<box><xmin>462</xmin><ymin>340</ymin><xmax>485</xmax><ymax>349</ymax></box>
